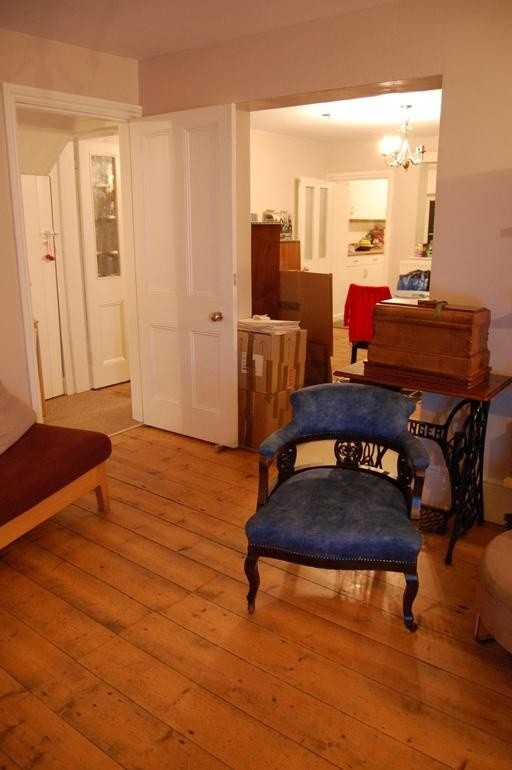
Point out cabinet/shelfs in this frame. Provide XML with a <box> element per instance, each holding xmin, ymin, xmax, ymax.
<box><xmin>349</xmin><ymin>252</ymin><xmax>383</xmax><ymax>285</ymax></box>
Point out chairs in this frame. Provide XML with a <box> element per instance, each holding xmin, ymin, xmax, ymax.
<box><xmin>0</xmin><ymin>383</ymin><xmax>111</xmax><ymax>550</ymax></box>
<box><xmin>349</xmin><ymin>284</ymin><xmax>392</xmax><ymax>363</ymax></box>
<box><xmin>399</xmin><ymin>270</ymin><xmax>430</xmax><ymax>291</ymax></box>
<box><xmin>244</xmin><ymin>382</ymin><xmax>421</xmax><ymax>634</ymax></box>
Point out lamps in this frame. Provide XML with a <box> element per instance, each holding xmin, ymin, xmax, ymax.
<box><xmin>377</xmin><ymin>104</ymin><xmax>426</xmax><ymax>171</ymax></box>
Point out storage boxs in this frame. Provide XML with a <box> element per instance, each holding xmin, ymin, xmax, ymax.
<box><xmin>237</xmin><ymin>270</ymin><xmax>333</xmax><ymax>449</ymax></box>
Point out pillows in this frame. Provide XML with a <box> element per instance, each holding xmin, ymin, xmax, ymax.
<box><xmin>0</xmin><ymin>384</ymin><xmax>36</xmax><ymax>455</ymax></box>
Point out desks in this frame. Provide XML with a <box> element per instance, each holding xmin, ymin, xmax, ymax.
<box><xmin>333</xmin><ymin>358</ymin><xmax>511</xmax><ymax>565</ymax></box>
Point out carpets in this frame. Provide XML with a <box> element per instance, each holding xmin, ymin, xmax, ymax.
<box><xmin>44</xmin><ymin>388</ymin><xmax>144</xmax><ymax>439</ymax></box>
